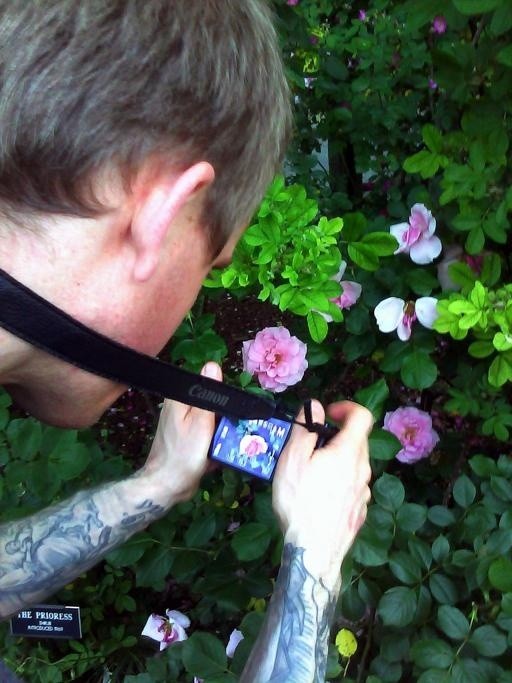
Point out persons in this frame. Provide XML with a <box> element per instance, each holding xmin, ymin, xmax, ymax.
<box><xmin>0</xmin><ymin>0</ymin><xmax>375</xmax><ymax>683</ymax></box>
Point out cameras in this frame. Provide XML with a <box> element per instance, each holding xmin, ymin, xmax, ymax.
<box><xmin>207</xmin><ymin>414</ymin><xmax>339</xmax><ymax>481</ymax></box>
